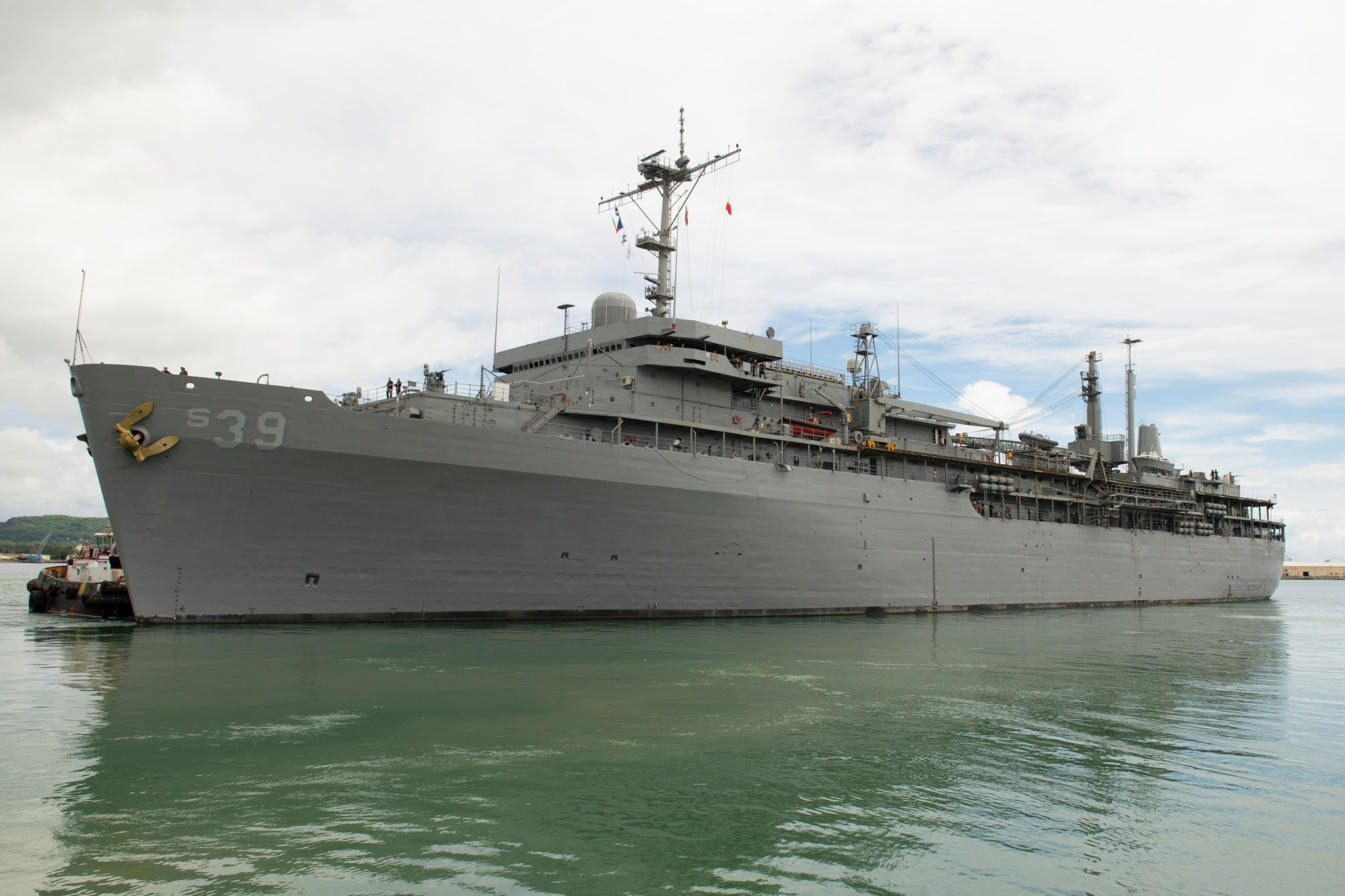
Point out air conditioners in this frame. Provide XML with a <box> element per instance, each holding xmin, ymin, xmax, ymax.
<box><xmin>940</xmin><ymin>438</ymin><xmax>947</xmax><ymax>444</ymax></box>
<box><xmin>622</xmin><ymin>377</ymin><xmax>631</xmax><ymax>386</ymax></box>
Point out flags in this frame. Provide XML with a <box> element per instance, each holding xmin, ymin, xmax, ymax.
<box><xmin>616</xmin><ymin>218</ymin><xmax>623</xmax><ymax>234</ymax></box>
<box><xmin>615</xmin><ymin>205</ymin><xmax>619</xmax><ymax>216</ymax></box>
<box><xmin>725</xmin><ymin>197</ymin><xmax>732</xmax><ymax>216</ymax></box>
<box><xmin>685</xmin><ymin>206</ymin><xmax>688</xmax><ymax>226</ymax></box>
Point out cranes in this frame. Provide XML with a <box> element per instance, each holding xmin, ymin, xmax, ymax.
<box><xmin>27</xmin><ymin>532</ymin><xmax>52</xmax><ymax>560</ymax></box>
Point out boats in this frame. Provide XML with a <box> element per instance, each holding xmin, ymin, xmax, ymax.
<box><xmin>27</xmin><ymin>521</ymin><xmax>134</xmax><ymax>621</ymax></box>
<box><xmin>69</xmin><ymin>105</ymin><xmax>1285</xmax><ymax>626</ymax></box>
<box><xmin>1018</xmin><ymin>430</ymin><xmax>1058</xmax><ymax>449</ymax></box>
<box><xmin>1131</xmin><ymin>443</ymin><xmax>1174</xmax><ymax>472</ymax></box>
<box><xmin>776</xmin><ymin>416</ymin><xmax>837</xmax><ymax>441</ymax></box>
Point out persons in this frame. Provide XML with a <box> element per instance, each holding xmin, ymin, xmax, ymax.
<box><xmin>1210</xmin><ymin>470</ymin><xmax>1214</xmax><ymax>480</ymax></box>
<box><xmin>978</xmin><ymin>501</ymin><xmax>994</xmax><ymax>518</ymax></box>
<box><xmin>665</xmin><ymin>343</ymin><xmax>671</xmax><ymax>351</ymax></box>
<box><xmin>386</xmin><ymin>377</ymin><xmax>394</xmax><ymax>399</ymax></box>
<box><xmin>729</xmin><ymin>354</ymin><xmax>740</xmax><ymax>368</ymax></box>
<box><xmin>656</xmin><ymin>341</ymin><xmax>661</xmax><ymax>351</ymax></box>
<box><xmin>1276</xmin><ymin>533</ymin><xmax>1282</xmax><ymax>541</ymax></box>
<box><xmin>1232</xmin><ymin>525</ymin><xmax>1241</xmax><ymax>537</ymax></box>
<box><xmin>1189</xmin><ymin>469</ymin><xmax>1193</xmax><ymax>478</ymax></box>
<box><xmin>1038</xmin><ymin>516</ymin><xmax>1043</xmax><ymax>521</ymax></box>
<box><xmin>1269</xmin><ymin>530</ymin><xmax>1274</xmax><ymax>540</ymax></box>
<box><xmin>1027</xmin><ymin>508</ymin><xmax>1032</xmax><ymax>521</ymax></box>
<box><xmin>395</xmin><ymin>379</ymin><xmax>402</xmax><ymax>396</ymax></box>
<box><xmin>758</xmin><ymin>361</ymin><xmax>767</xmax><ymax>379</ymax></box>
<box><xmin>1214</xmin><ymin>469</ymin><xmax>1218</xmax><ymax>480</ymax></box>
<box><xmin>744</xmin><ymin>357</ymin><xmax>759</xmax><ymax>377</ymax></box>
